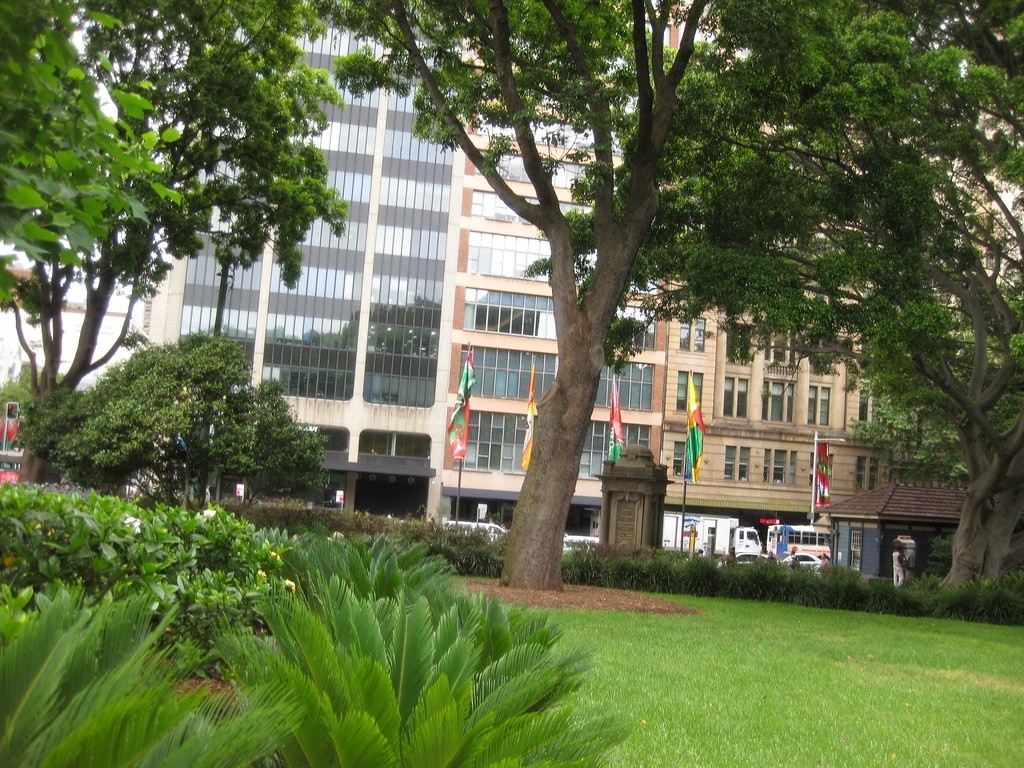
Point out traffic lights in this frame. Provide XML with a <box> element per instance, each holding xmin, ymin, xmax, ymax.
<box><xmin>5</xmin><ymin>402</ymin><xmax>20</xmax><ymax>420</ymax></box>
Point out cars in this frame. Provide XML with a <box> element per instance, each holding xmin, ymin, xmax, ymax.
<box><xmin>783</xmin><ymin>552</ymin><xmax>822</xmax><ymax>565</ymax></box>
<box><xmin>562</xmin><ymin>535</ymin><xmax>600</xmax><ymax>551</ymax></box>
<box><xmin>736</xmin><ymin>553</ymin><xmax>769</xmax><ymax>564</ymax></box>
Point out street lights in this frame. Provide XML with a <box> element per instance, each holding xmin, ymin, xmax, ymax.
<box><xmin>810</xmin><ymin>430</ymin><xmax>846</xmax><ymax>524</ymax></box>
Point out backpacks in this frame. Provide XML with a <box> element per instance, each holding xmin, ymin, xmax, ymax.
<box><xmin>792</xmin><ymin>557</ymin><xmax>799</xmax><ymax>568</ymax></box>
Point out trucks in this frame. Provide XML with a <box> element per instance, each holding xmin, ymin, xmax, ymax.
<box><xmin>661</xmin><ymin>509</ymin><xmax>763</xmax><ymax>558</ymax></box>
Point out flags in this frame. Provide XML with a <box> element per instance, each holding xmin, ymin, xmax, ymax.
<box><xmin>520</xmin><ymin>355</ymin><xmax>538</xmax><ymax>473</ymax></box>
<box><xmin>685</xmin><ymin>371</ymin><xmax>706</xmax><ymax>487</ymax></box>
<box><xmin>602</xmin><ymin>369</ymin><xmax>625</xmax><ymax>466</ymax></box>
<box><xmin>447</xmin><ymin>349</ymin><xmax>478</xmax><ymax>464</ymax></box>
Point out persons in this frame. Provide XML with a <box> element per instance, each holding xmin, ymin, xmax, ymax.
<box><xmin>892</xmin><ymin>546</ymin><xmax>906</xmax><ymax>587</ymax></box>
<box><xmin>723</xmin><ymin>550</ymin><xmax>738</xmax><ymax>566</ymax></box>
<box><xmin>819</xmin><ymin>552</ymin><xmax>830</xmax><ymax>569</ymax></box>
<box><xmin>698</xmin><ymin>549</ymin><xmax>706</xmax><ymax>558</ymax></box>
<box><xmin>767</xmin><ymin>551</ymin><xmax>776</xmax><ymax>565</ymax></box>
<box><xmin>787</xmin><ymin>550</ymin><xmax>800</xmax><ymax>569</ymax></box>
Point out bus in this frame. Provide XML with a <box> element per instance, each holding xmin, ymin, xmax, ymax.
<box><xmin>766</xmin><ymin>524</ymin><xmax>831</xmax><ymax>559</ymax></box>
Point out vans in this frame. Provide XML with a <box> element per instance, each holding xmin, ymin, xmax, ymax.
<box><xmin>443</xmin><ymin>520</ymin><xmax>506</xmax><ymax>542</ymax></box>
